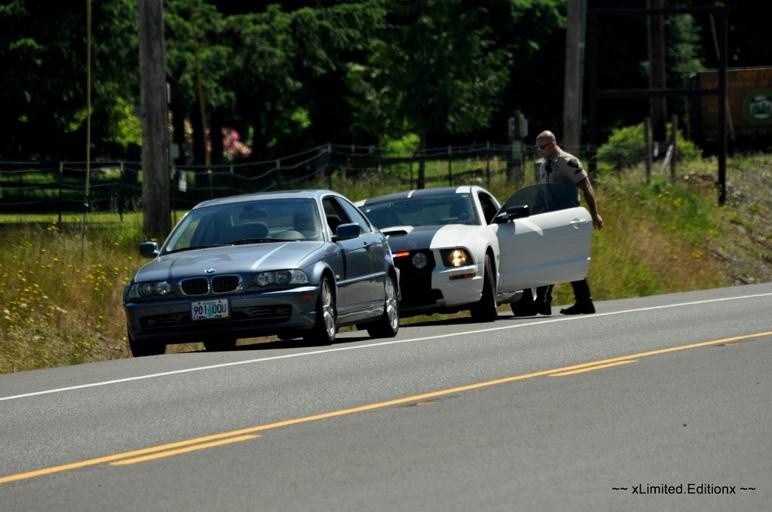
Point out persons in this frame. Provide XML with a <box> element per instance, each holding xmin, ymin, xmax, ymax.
<box><xmin>517</xmin><ymin>129</ymin><xmax>605</xmax><ymax>315</ymax></box>
<box><xmin>506</xmin><ymin>109</ymin><xmax>529</xmax><ymax>179</ymax></box>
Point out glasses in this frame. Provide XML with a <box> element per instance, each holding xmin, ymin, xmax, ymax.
<box><xmin>538</xmin><ymin>143</ymin><xmax>552</xmax><ymax>150</ymax></box>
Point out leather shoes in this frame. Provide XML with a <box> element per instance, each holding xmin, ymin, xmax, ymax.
<box><xmin>522</xmin><ymin>301</ymin><xmax>551</xmax><ymax>316</ymax></box>
<box><xmin>559</xmin><ymin>298</ymin><xmax>595</xmax><ymax>314</ymax></box>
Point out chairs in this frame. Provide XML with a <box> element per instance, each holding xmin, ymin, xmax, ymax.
<box><xmin>217</xmin><ymin>218</ymin><xmax>268</xmax><ymax>244</ymax></box>
<box><xmin>292</xmin><ymin>210</ymin><xmax>341</xmax><ymax>235</ymax></box>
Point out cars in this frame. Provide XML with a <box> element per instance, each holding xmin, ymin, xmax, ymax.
<box><xmin>123</xmin><ymin>183</ymin><xmax>593</xmax><ymax>357</ymax></box>
